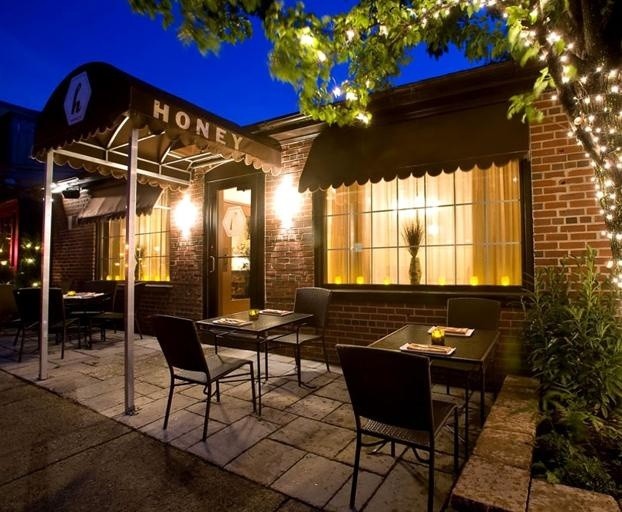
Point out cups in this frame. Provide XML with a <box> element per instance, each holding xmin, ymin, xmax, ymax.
<box><xmin>431</xmin><ymin>330</ymin><xmax>444</xmax><ymax>344</ymax></box>
<box><xmin>69</xmin><ymin>290</ymin><xmax>76</xmax><ymax>297</ymax></box>
<box><xmin>248</xmin><ymin>308</ymin><xmax>260</xmax><ymax>318</ymax></box>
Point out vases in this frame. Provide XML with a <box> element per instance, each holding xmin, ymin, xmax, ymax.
<box><xmin>408</xmin><ymin>256</ymin><xmax>421</xmax><ymax>284</ymax></box>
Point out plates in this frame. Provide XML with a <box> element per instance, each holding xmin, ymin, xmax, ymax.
<box><xmin>213</xmin><ymin>318</ymin><xmax>252</xmax><ymax>327</ymax></box>
<box><xmin>258</xmin><ymin>309</ymin><xmax>294</xmax><ymax>317</ymax></box>
<box><xmin>428</xmin><ymin>326</ymin><xmax>475</xmax><ymax>337</ymax></box>
<box><xmin>399</xmin><ymin>343</ymin><xmax>457</xmax><ymax>357</ymax></box>
